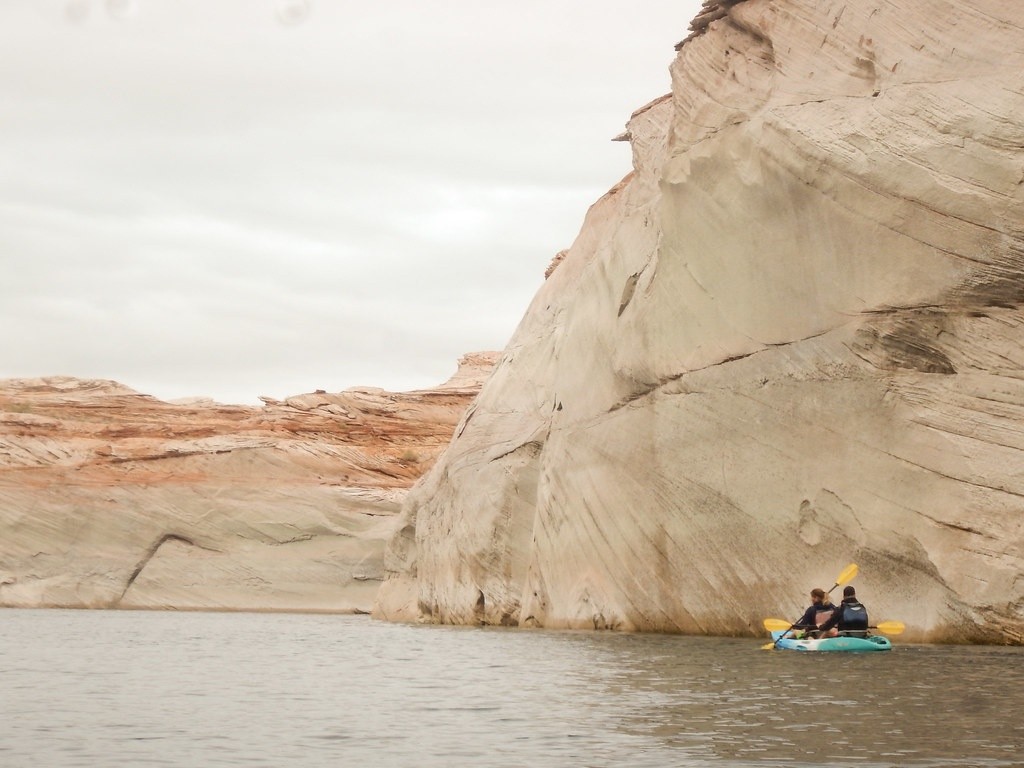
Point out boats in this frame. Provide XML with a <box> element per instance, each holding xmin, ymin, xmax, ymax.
<box><xmin>762</xmin><ymin>617</ymin><xmax>893</xmax><ymax>651</ymax></box>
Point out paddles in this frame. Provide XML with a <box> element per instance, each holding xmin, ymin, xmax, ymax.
<box><xmin>763</xmin><ymin>617</ymin><xmax>906</xmax><ymax>636</ymax></box>
<box><xmin>761</xmin><ymin>562</ymin><xmax>860</xmax><ymax>650</ymax></box>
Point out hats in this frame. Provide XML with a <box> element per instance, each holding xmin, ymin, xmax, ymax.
<box><xmin>844</xmin><ymin>586</ymin><xmax>855</xmax><ymax>596</ymax></box>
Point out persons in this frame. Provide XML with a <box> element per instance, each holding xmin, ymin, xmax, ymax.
<box><xmin>782</xmin><ymin>588</ymin><xmax>837</xmax><ymax>639</ymax></box>
<box><xmin>816</xmin><ymin>585</ymin><xmax>868</xmax><ymax>639</ymax></box>
